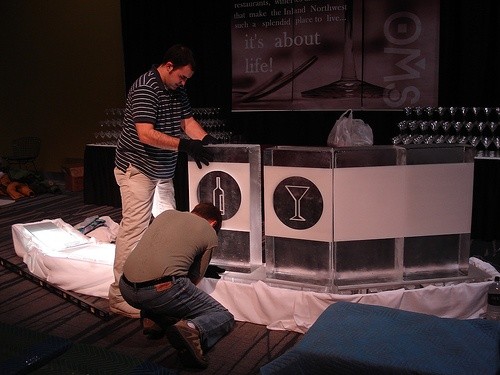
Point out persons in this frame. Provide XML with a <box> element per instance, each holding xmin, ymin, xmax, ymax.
<box><xmin>108</xmin><ymin>45</ymin><xmax>218</xmax><ymax>319</ymax></box>
<box><xmin>119</xmin><ymin>202</ymin><xmax>236</xmax><ymax>369</ymax></box>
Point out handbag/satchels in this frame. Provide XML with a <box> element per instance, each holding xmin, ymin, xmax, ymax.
<box><xmin>327</xmin><ymin>109</ymin><xmax>373</xmax><ymax>146</ymax></box>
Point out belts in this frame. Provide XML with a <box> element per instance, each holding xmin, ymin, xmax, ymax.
<box><xmin>121</xmin><ymin>272</ymin><xmax>184</xmax><ymax>288</ymax></box>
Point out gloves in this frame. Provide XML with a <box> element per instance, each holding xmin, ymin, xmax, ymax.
<box><xmin>202</xmin><ymin>135</ymin><xmax>220</xmax><ymax>144</ymax></box>
<box><xmin>204</xmin><ymin>264</ymin><xmax>226</xmax><ymax>279</ymax></box>
<box><xmin>178</xmin><ymin>138</ymin><xmax>216</xmax><ymax>169</ymax></box>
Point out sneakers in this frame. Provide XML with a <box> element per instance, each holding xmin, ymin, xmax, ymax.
<box><xmin>110</xmin><ymin>301</ymin><xmax>140</xmax><ymax>319</ymax></box>
<box><xmin>169</xmin><ymin>320</ymin><xmax>209</xmax><ymax>370</ymax></box>
<box><xmin>143</xmin><ymin>318</ymin><xmax>159</xmax><ymax>335</ymax></box>
